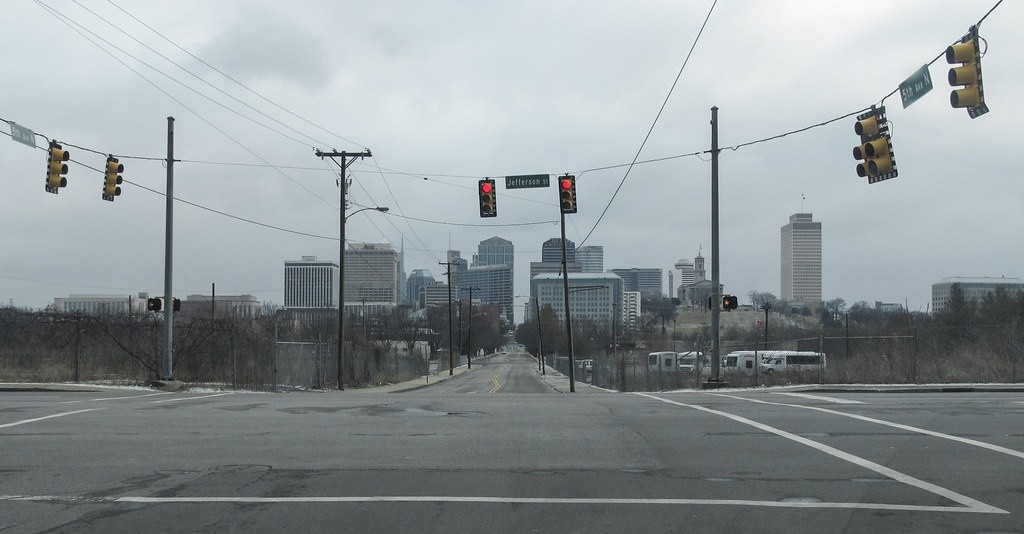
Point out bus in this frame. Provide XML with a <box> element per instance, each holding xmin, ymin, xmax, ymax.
<box><xmin>648</xmin><ymin>350</ymin><xmax>826</xmax><ymax>376</ymax></box>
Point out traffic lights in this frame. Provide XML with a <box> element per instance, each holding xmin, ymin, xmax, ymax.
<box><xmin>148</xmin><ymin>298</ymin><xmax>161</xmax><ymax>312</ymax></box>
<box><xmin>105</xmin><ymin>161</ymin><xmax>125</xmax><ymax>197</ymax></box>
<box><xmin>853</xmin><ymin>114</ymin><xmax>892</xmax><ymax>178</ymax></box>
<box><xmin>558</xmin><ymin>175</ymin><xmax>578</xmax><ymax>213</ymax></box>
<box><xmin>946</xmin><ymin>41</ymin><xmax>980</xmax><ymax>110</ymax></box>
<box><xmin>723</xmin><ymin>295</ymin><xmax>737</xmax><ymax>310</ymax></box>
<box><xmin>478</xmin><ymin>179</ymin><xmax>497</xmax><ymax>218</ymax></box>
<box><xmin>50</xmin><ymin>148</ymin><xmax>70</xmax><ymax>189</ymax></box>
<box><xmin>174</xmin><ymin>298</ymin><xmax>181</xmax><ymax>312</ymax></box>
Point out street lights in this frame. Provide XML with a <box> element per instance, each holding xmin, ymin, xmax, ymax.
<box><xmin>515</xmin><ymin>295</ymin><xmax>546</xmax><ymax>373</ymax></box>
<box><xmin>524</xmin><ymin>301</ymin><xmax>541</xmax><ymax>371</ymax></box>
<box><xmin>337</xmin><ymin>206</ymin><xmax>390</xmax><ymax>388</ymax></box>
